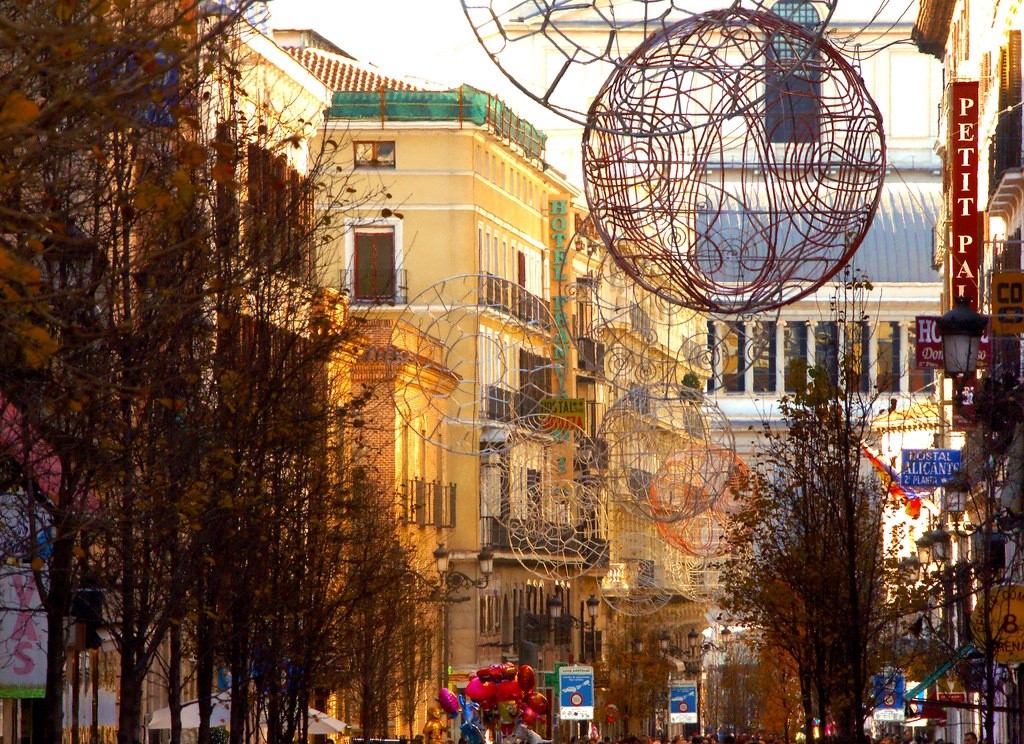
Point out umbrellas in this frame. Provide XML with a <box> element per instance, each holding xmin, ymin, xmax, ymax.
<box><xmin>150</xmin><ymin>680</ymin><xmax>347</xmax><ymax>734</ymax></box>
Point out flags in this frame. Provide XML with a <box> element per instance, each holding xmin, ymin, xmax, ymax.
<box><xmin>862</xmin><ymin>444</ymin><xmax>921</xmax><ymax>519</ymax></box>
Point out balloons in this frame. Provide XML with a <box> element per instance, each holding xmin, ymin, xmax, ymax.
<box><xmin>439</xmin><ymin>688</ymin><xmax>459</xmax><ymax>713</ymax></box>
<box><xmin>459</xmin><ymin>661</ymin><xmax>554</xmax><ymax>744</ymax></box>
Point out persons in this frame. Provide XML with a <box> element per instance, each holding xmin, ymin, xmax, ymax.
<box><xmin>725</xmin><ymin>729</ymin><xmax>735</xmax><ymax>737</ymax></box>
<box><xmin>423</xmin><ymin>707</ymin><xmax>452</xmax><ymax>744</ymax></box>
<box><xmin>814</xmin><ymin>730</ymin><xmax>987</xmax><ymax>744</ymax></box>
<box><xmin>619</xmin><ymin>734</ymin><xmax>766</xmax><ymax>744</ymax></box>
<box><xmin>570</xmin><ymin>736</ymin><xmax>611</xmax><ymax>744</ymax></box>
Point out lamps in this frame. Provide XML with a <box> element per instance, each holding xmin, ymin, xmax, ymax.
<box><xmin>565</xmin><ymin>593</ymin><xmax>600</xmax><ymax>631</ymax></box>
<box><xmin>637</xmin><ymin>632</ymin><xmax>668</xmax><ymax>662</ymax></box>
<box><xmin>447</xmin><ymin>545</ymin><xmax>497</xmax><ymax>593</ymax></box>
<box><xmin>944</xmin><ymin>471</ymin><xmax>995</xmax><ymax>537</ymax></box>
<box><xmin>521</xmin><ymin>595</ymin><xmax>563</xmax><ymax>632</ymax></box>
<box><xmin>699</xmin><ymin>625</ymin><xmax>733</xmax><ymax>653</ymax></box>
<box><xmin>916</xmin><ymin>535</ymin><xmax>934</xmax><ymax>585</ymax></box>
<box><xmin>398</xmin><ymin>542</ymin><xmax>454</xmax><ymax>590</ymax></box>
<box><xmin>907</xmin><ymin>614</ymin><xmax>1010</xmax><ymax>700</ymax></box>
<box><xmin>670</xmin><ymin>628</ymin><xmax>698</xmax><ymax>656</ymax></box>
<box><xmin>932</xmin><ymin>523</ymin><xmax>971</xmax><ymax>582</ymax></box>
<box><xmin>939</xmin><ymin>290</ymin><xmax>1016</xmax><ymax>446</ymax></box>
